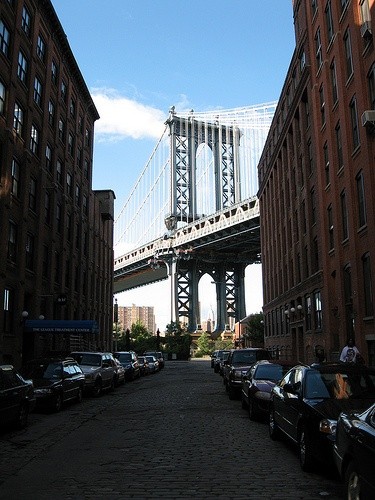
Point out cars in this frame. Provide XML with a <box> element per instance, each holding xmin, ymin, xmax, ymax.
<box><xmin>0</xmin><ymin>357</ymin><xmax>86</xmax><ymax>423</ymax></box>
<box><xmin>242</xmin><ymin>360</ymin><xmax>305</xmax><ymax>417</ymax></box>
<box><xmin>268</xmin><ymin>362</ymin><xmax>375</xmax><ymax>500</ymax></box>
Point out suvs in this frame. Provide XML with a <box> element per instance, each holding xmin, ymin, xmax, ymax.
<box><xmin>210</xmin><ymin>348</ymin><xmax>275</xmax><ymax>400</ymax></box>
<box><xmin>113</xmin><ymin>350</ymin><xmax>165</xmax><ymax>386</ymax></box>
<box><xmin>69</xmin><ymin>352</ymin><xmax>118</xmax><ymax>398</ymax></box>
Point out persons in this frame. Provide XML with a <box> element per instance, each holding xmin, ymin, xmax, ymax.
<box><xmin>307</xmin><ymin>345</ymin><xmax>328</xmax><ymax>366</ymax></box>
<box><xmin>339</xmin><ymin>336</ymin><xmax>365</xmax><ymax>364</ymax></box>
